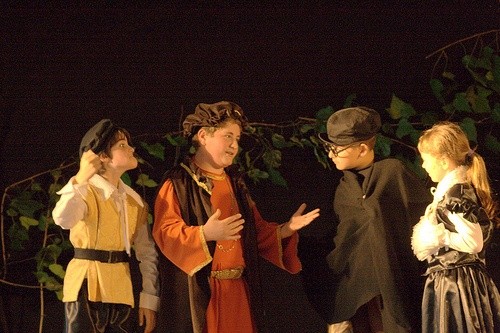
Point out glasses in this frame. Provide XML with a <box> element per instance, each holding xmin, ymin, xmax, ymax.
<box><xmin>324</xmin><ymin>143</ymin><xmax>356</xmax><ymax>157</ymax></box>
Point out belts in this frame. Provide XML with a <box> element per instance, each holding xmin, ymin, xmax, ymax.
<box><xmin>210</xmin><ymin>266</ymin><xmax>244</xmax><ymax>280</ymax></box>
<box><xmin>73</xmin><ymin>248</ymin><xmax>143</xmax><ymax>319</ymax></box>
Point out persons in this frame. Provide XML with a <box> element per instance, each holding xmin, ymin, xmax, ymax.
<box><xmin>297</xmin><ymin>108</ymin><xmax>434</xmax><ymax>333</ymax></box>
<box><xmin>50</xmin><ymin>118</ymin><xmax>164</xmax><ymax>333</ymax></box>
<box><xmin>153</xmin><ymin>101</ymin><xmax>322</xmax><ymax>333</ymax></box>
<box><xmin>410</xmin><ymin>121</ymin><xmax>500</xmax><ymax>333</ymax></box>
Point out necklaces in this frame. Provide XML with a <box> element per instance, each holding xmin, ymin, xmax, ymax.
<box><xmin>198</xmin><ymin>174</ymin><xmax>237</xmax><ymax>252</ymax></box>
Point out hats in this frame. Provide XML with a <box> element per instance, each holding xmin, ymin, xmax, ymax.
<box><xmin>79</xmin><ymin>118</ymin><xmax>114</xmax><ymax>161</ymax></box>
<box><xmin>182</xmin><ymin>101</ymin><xmax>248</xmax><ymax>139</ymax></box>
<box><xmin>318</xmin><ymin>106</ymin><xmax>382</xmax><ymax>146</ymax></box>
